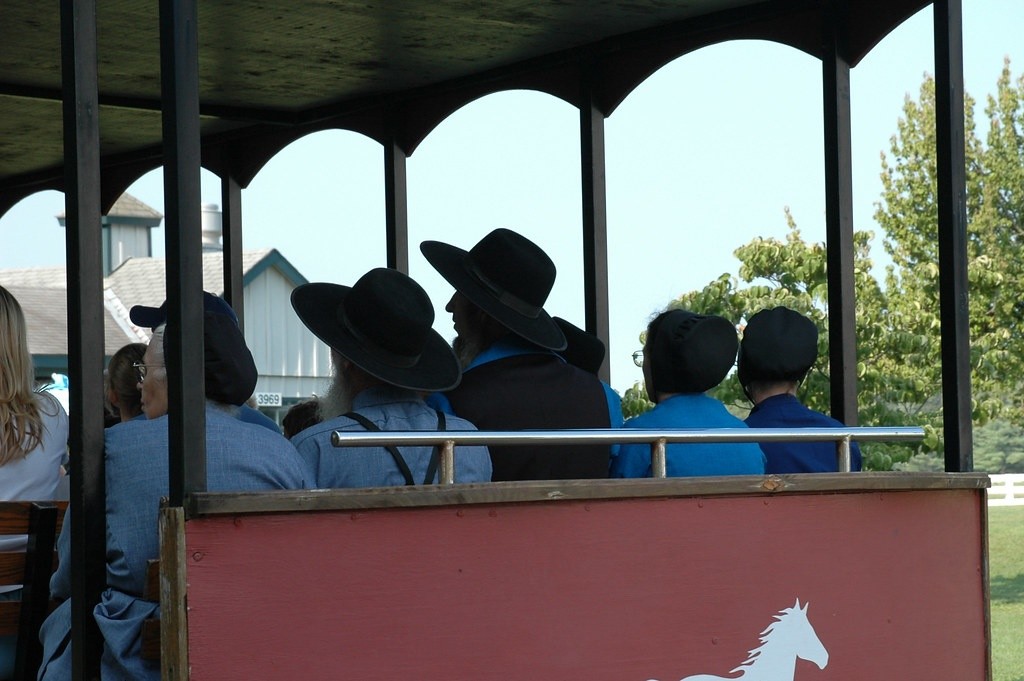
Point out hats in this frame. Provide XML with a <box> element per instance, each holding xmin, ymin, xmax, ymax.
<box><xmin>657</xmin><ymin>309</ymin><xmax>739</xmax><ymax>392</ymax></box>
<box><xmin>290</xmin><ymin>268</ymin><xmax>461</xmax><ymax>392</ymax></box>
<box><xmin>129</xmin><ymin>292</ymin><xmax>258</xmax><ymax>406</ymax></box>
<box><xmin>552</xmin><ymin>317</ymin><xmax>606</xmax><ymax>375</ymax></box>
<box><xmin>739</xmin><ymin>306</ymin><xmax>820</xmax><ymax>379</ymax></box>
<box><xmin>419</xmin><ymin>228</ymin><xmax>568</xmax><ymax>350</ymax></box>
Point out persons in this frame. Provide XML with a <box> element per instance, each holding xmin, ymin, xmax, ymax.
<box><xmin>39</xmin><ymin>325</ymin><xmax>317</xmax><ymax>681</ymax></box>
<box><xmin>608</xmin><ymin>309</ymin><xmax>767</xmax><ymax>477</ymax></box>
<box><xmin>103</xmin><ymin>343</ymin><xmax>147</xmax><ymax>429</ymax></box>
<box><xmin>129</xmin><ymin>293</ymin><xmax>282</xmax><ymax>433</ymax></box>
<box><xmin>551</xmin><ymin>316</ymin><xmax>622</xmax><ymax>457</ymax></box>
<box><xmin>420</xmin><ymin>228</ymin><xmax>611</xmax><ymax>480</ymax></box>
<box><xmin>288</xmin><ymin>268</ymin><xmax>493</xmax><ymax>488</ymax></box>
<box><xmin>738</xmin><ymin>306</ymin><xmax>862</xmax><ymax>474</ymax></box>
<box><xmin>0</xmin><ymin>285</ymin><xmax>69</xmax><ymax>681</ymax></box>
<box><xmin>282</xmin><ymin>400</ymin><xmax>324</xmax><ymax>440</ymax></box>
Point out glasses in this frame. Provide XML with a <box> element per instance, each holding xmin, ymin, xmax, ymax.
<box><xmin>632</xmin><ymin>350</ymin><xmax>651</xmax><ymax>368</ymax></box>
<box><xmin>132</xmin><ymin>360</ymin><xmax>167</xmax><ymax>383</ymax></box>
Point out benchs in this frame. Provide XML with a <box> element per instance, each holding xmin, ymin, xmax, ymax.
<box><xmin>0</xmin><ymin>499</ymin><xmax>69</xmax><ymax>681</ymax></box>
<box><xmin>141</xmin><ymin>426</ymin><xmax>995</xmax><ymax>679</ymax></box>
